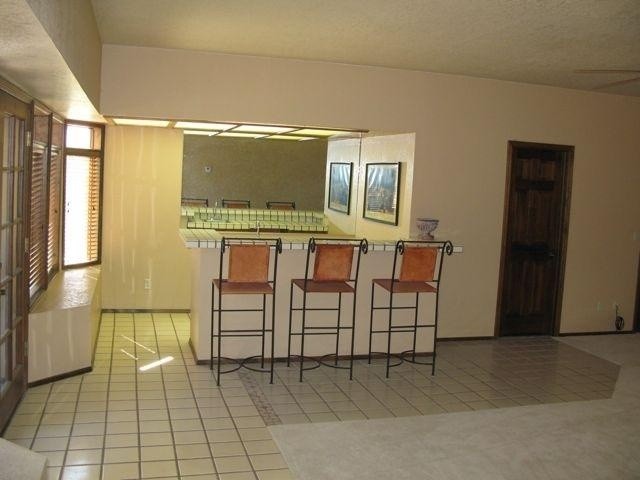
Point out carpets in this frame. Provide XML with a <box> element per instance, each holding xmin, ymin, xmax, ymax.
<box><xmin>266</xmin><ymin>332</ymin><xmax>640</xmax><ymax>480</ymax></box>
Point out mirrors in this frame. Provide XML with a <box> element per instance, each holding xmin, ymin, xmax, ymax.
<box><xmin>181</xmin><ymin>131</ymin><xmax>361</xmax><ymax>236</ymax></box>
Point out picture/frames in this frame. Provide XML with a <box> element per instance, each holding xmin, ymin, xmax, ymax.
<box><xmin>328</xmin><ymin>161</ymin><xmax>353</xmax><ymax>215</ymax></box>
<box><xmin>362</xmin><ymin>161</ymin><xmax>401</xmax><ymax>226</ymax></box>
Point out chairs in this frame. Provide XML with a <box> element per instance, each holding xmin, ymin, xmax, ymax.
<box><xmin>210</xmin><ymin>237</ymin><xmax>282</xmax><ymax>385</ymax></box>
<box><xmin>287</xmin><ymin>237</ymin><xmax>369</xmax><ymax>382</ymax></box>
<box><xmin>266</xmin><ymin>201</ymin><xmax>295</xmax><ymax>210</ymax></box>
<box><xmin>222</xmin><ymin>197</ymin><xmax>250</xmax><ymax>209</ymax></box>
<box><xmin>181</xmin><ymin>198</ymin><xmax>208</xmax><ymax>207</ymax></box>
<box><xmin>369</xmin><ymin>239</ymin><xmax>453</xmax><ymax>378</ymax></box>
